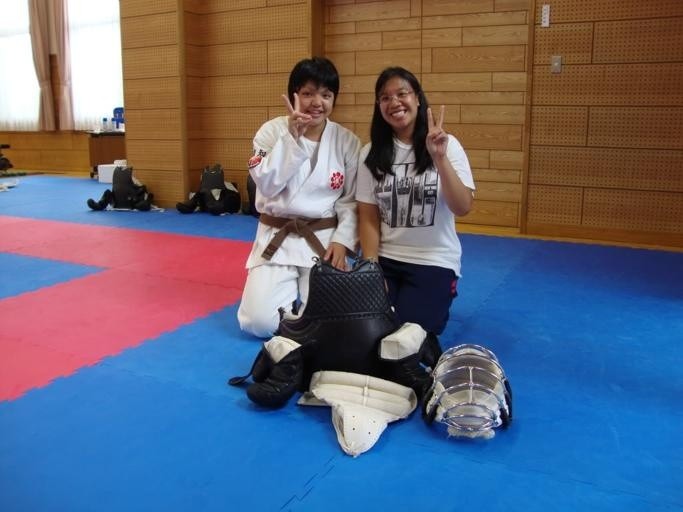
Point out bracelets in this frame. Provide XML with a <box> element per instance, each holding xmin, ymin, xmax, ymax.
<box><xmin>362</xmin><ymin>255</ymin><xmax>380</xmax><ymax>262</ymax></box>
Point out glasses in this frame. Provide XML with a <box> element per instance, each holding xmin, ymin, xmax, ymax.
<box><xmin>375</xmin><ymin>89</ymin><xmax>415</xmax><ymax>102</ymax></box>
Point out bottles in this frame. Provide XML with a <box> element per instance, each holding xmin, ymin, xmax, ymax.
<box><xmin>102</xmin><ymin>118</ymin><xmax>108</xmax><ymax>132</ymax></box>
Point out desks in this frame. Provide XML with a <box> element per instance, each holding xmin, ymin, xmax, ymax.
<box><xmin>86</xmin><ymin>129</ymin><xmax>125</xmax><ymax>178</ymax></box>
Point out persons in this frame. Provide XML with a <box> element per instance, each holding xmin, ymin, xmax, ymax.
<box><xmin>352</xmin><ymin>62</ymin><xmax>478</xmax><ymax>336</ymax></box>
<box><xmin>233</xmin><ymin>55</ymin><xmax>365</xmax><ymax>343</ymax></box>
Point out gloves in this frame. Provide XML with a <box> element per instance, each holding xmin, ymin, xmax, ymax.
<box><xmin>86</xmin><ymin>188</ymin><xmax>112</xmax><ymax>211</ymax></box>
<box><xmin>246</xmin><ymin>334</ymin><xmax>314</xmax><ymax>412</ymax></box>
<box><xmin>130</xmin><ymin>193</ymin><xmax>154</xmax><ymax>212</ymax></box>
<box><xmin>175</xmin><ymin>189</ymin><xmax>241</xmax><ymax>215</ymax></box>
